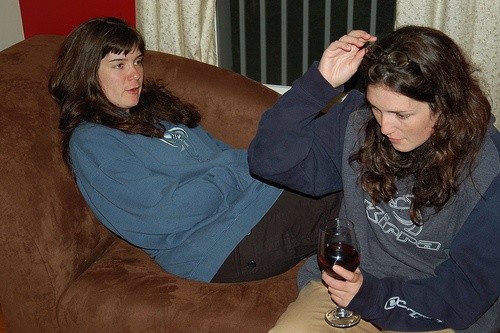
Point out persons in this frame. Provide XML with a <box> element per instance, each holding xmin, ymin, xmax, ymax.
<box><xmin>247</xmin><ymin>24</ymin><xmax>500</xmax><ymax>333</ymax></box>
<box><xmin>47</xmin><ymin>16</ymin><xmax>345</xmax><ymax>284</ymax></box>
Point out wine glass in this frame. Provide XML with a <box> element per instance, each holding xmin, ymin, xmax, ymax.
<box><xmin>317</xmin><ymin>218</ymin><xmax>361</xmax><ymax>328</ymax></box>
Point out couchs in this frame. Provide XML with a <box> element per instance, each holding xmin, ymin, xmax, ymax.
<box><xmin>0</xmin><ymin>34</ymin><xmax>315</xmax><ymax>333</ymax></box>
